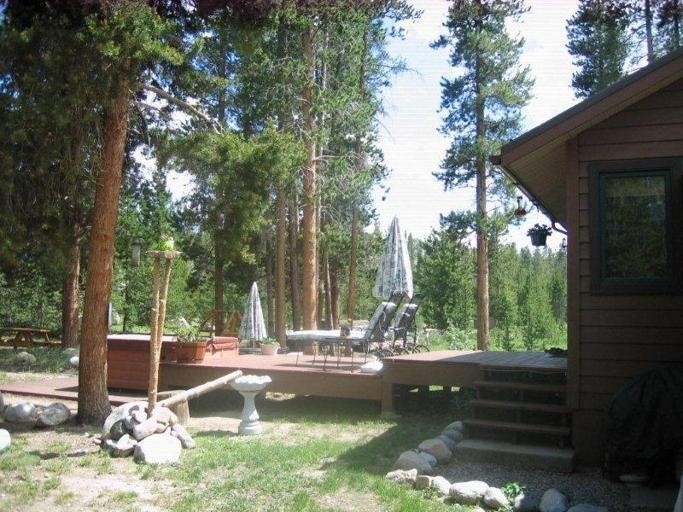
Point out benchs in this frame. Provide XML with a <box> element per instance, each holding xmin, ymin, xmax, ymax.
<box><xmin>0</xmin><ymin>327</ymin><xmax>51</xmax><ymax>350</ymax></box>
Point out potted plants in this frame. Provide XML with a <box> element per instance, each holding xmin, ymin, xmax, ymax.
<box><xmin>527</xmin><ymin>224</ymin><xmax>552</xmax><ymax>246</ymax></box>
<box><xmin>174</xmin><ymin>318</ymin><xmax>205</xmax><ymax>363</ymax></box>
<box><xmin>257</xmin><ymin>337</ymin><xmax>281</xmax><ymax>355</ymax></box>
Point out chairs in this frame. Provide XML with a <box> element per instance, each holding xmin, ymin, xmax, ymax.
<box><xmin>340</xmin><ymin>294</ymin><xmax>426</xmax><ymax>361</ymax></box>
<box><xmin>288</xmin><ymin>291</ymin><xmax>405</xmax><ymax>368</ymax></box>
